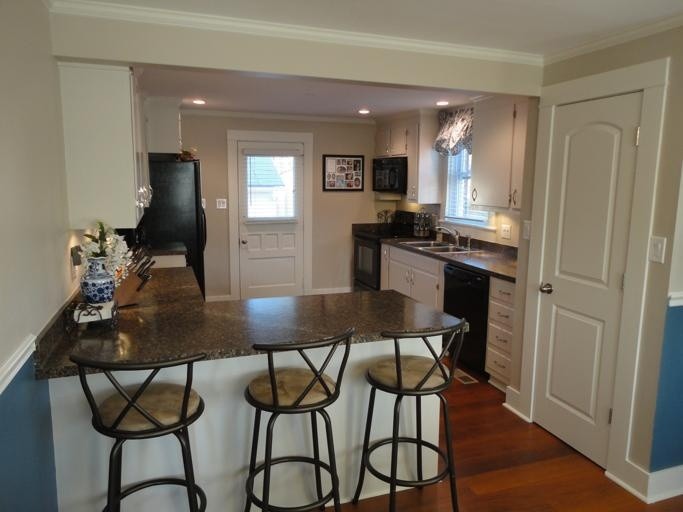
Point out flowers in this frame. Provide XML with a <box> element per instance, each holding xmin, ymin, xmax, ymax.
<box><xmin>77</xmin><ymin>220</ymin><xmax>134</xmax><ymax>287</ymax></box>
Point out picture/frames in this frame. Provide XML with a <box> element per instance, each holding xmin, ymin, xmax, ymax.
<box><xmin>322</xmin><ymin>154</ymin><xmax>365</xmax><ymax>192</ymax></box>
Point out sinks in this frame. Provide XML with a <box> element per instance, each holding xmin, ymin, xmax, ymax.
<box><xmin>420</xmin><ymin>245</ymin><xmax>485</xmax><ymax>254</ymax></box>
<box><xmin>398</xmin><ymin>240</ymin><xmax>455</xmax><ymax>248</ymax></box>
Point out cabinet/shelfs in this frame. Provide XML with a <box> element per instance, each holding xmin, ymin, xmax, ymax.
<box><xmin>468</xmin><ymin>102</ymin><xmax>515</xmax><ymax>209</ymax></box>
<box><xmin>406</xmin><ymin>115</ymin><xmax>442</xmax><ymax>206</ymax></box>
<box><xmin>56</xmin><ymin>59</ymin><xmax>151</xmax><ymax>230</ymax></box>
<box><xmin>484</xmin><ymin>273</ymin><xmax>515</xmax><ymax>395</ymax></box>
<box><xmin>374</xmin><ymin>125</ymin><xmax>407</xmax><ymax>157</ymax></box>
<box><xmin>380</xmin><ymin>243</ymin><xmax>439</xmax><ymax>308</ymax></box>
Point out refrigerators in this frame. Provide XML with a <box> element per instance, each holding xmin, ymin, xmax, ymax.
<box><xmin>135</xmin><ymin>159</ymin><xmax>206</xmax><ymax>300</ymax></box>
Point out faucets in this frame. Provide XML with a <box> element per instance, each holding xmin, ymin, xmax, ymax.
<box><xmin>435</xmin><ymin>224</ymin><xmax>461</xmax><ymax>247</ymax></box>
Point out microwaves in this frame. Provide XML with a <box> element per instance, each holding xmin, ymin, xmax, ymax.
<box><xmin>373</xmin><ymin>157</ymin><xmax>408</xmax><ymax>193</ymax></box>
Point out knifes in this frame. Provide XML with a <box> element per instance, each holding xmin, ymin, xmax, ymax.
<box><xmin>129</xmin><ymin>247</ymin><xmax>155</xmax><ymax>292</ymax></box>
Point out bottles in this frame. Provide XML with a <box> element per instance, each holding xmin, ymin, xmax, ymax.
<box><xmin>415</xmin><ymin>208</ymin><xmax>430</xmax><ymax>231</ymax></box>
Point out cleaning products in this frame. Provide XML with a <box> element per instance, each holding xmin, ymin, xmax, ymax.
<box><xmin>436</xmin><ymin>221</ymin><xmax>443</xmax><ymax>243</ymax></box>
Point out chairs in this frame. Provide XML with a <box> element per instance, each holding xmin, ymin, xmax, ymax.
<box><xmin>68</xmin><ymin>352</ymin><xmax>208</xmax><ymax>512</ymax></box>
<box><xmin>352</xmin><ymin>318</ymin><xmax>467</xmax><ymax>512</ymax></box>
<box><xmin>244</xmin><ymin>327</ymin><xmax>356</xmax><ymax>512</ymax></box>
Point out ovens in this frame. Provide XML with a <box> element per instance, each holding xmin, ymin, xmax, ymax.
<box><xmin>354</xmin><ymin>239</ymin><xmax>381</xmax><ymax>291</ymax></box>
<box><xmin>442</xmin><ymin>266</ymin><xmax>489</xmax><ymax>376</ymax></box>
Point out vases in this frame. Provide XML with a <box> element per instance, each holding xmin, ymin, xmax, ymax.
<box><xmin>78</xmin><ymin>256</ymin><xmax>115</xmax><ymax>302</ymax></box>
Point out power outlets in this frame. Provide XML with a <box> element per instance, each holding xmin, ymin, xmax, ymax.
<box><xmin>500</xmin><ymin>224</ymin><xmax>512</xmax><ymax>240</ymax></box>
